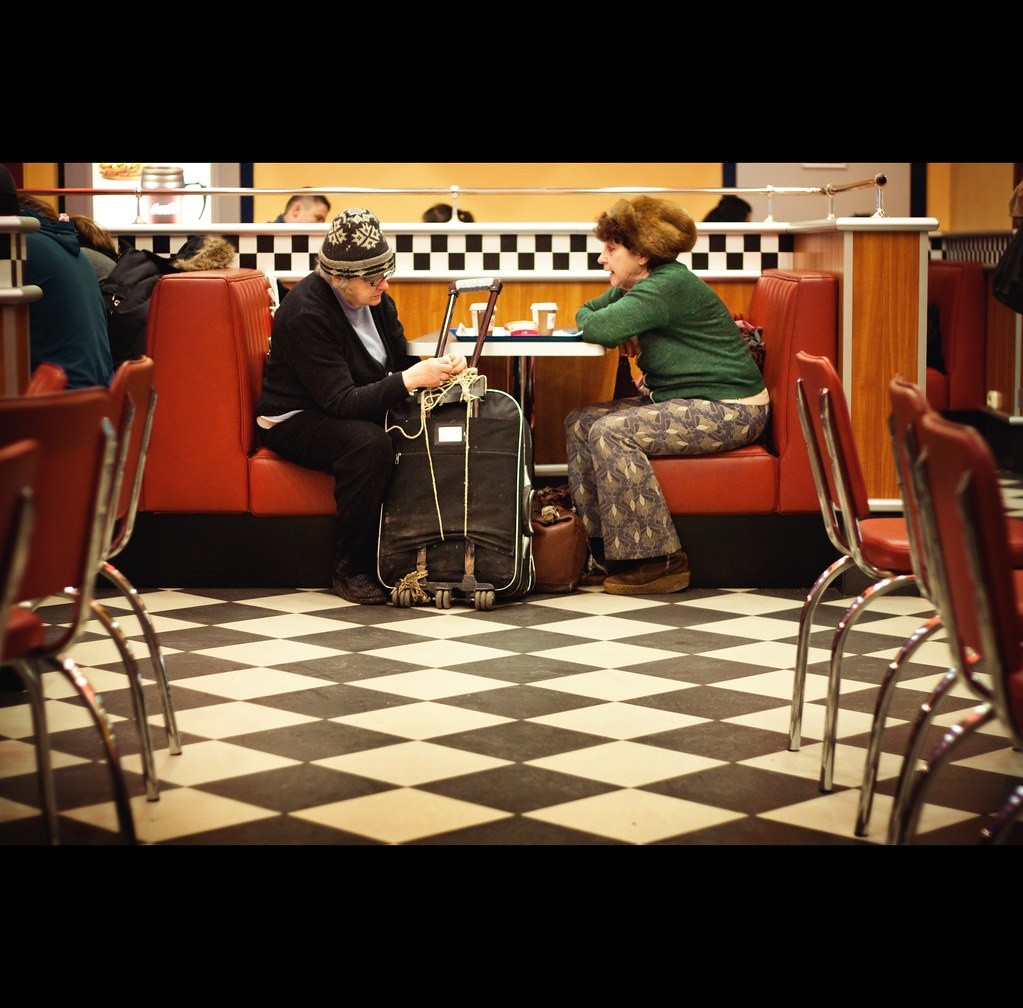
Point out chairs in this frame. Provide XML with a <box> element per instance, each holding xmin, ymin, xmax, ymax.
<box><xmin>787</xmin><ymin>351</ymin><xmax>1023</xmax><ymax>843</ymax></box>
<box><xmin>0</xmin><ymin>353</ymin><xmax>180</xmax><ymax>846</ymax></box>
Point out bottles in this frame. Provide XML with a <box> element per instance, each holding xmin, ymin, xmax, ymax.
<box><xmin>134</xmin><ymin>168</ymin><xmax>188</xmax><ymax>228</ymax></box>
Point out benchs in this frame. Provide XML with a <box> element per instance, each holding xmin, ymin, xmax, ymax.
<box><xmin>93</xmin><ymin>268</ymin><xmax>339</xmax><ymax>583</ymax></box>
<box><xmin>927</xmin><ymin>258</ymin><xmax>986</xmax><ymax>412</ymax></box>
<box><xmin>647</xmin><ymin>269</ymin><xmax>840</xmax><ymax>588</ymax></box>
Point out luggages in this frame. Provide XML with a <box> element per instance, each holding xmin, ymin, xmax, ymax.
<box><xmin>378</xmin><ymin>278</ymin><xmax>536</xmax><ymax>610</ymax></box>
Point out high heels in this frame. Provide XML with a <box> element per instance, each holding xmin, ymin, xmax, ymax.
<box><xmin>581</xmin><ymin>557</ymin><xmax>620</xmax><ymax>585</ymax></box>
<box><xmin>602</xmin><ymin>548</ymin><xmax>691</xmax><ymax>596</ymax></box>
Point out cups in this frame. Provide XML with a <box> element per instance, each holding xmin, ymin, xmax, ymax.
<box><xmin>469</xmin><ymin>303</ymin><xmax>497</xmax><ymax>338</ymax></box>
<box><xmin>530</xmin><ymin>302</ymin><xmax>558</xmax><ymax>337</ymax></box>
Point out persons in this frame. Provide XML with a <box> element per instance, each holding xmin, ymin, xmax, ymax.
<box><xmin>267</xmin><ymin>186</ymin><xmax>331</xmax><ymax>223</ymax></box>
<box><xmin>16</xmin><ymin>194</ymin><xmax>115</xmax><ymax>393</ymax></box>
<box><xmin>562</xmin><ymin>195</ymin><xmax>771</xmax><ymax>593</ymax></box>
<box><xmin>422</xmin><ymin>204</ymin><xmax>473</xmax><ymax>223</ymax></box>
<box><xmin>702</xmin><ymin>195</ymin><xmax>752</xmax><ymax>223</ymax></box>
<box><xmin>59</xmin><ymin>211</ymin><xmax>117</xmax><ymax>277</ymax></box>
<box><xmin>256</xmin><ymin>210</ymin><xmax>468</xmax><ymax>605</ymax></box>
<box><xmin>0</xmin><ymin>164</ymin><xmax>23</xmax><ymax>261</ymax></box>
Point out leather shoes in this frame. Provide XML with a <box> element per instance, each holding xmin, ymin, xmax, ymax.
<box><xmin>332</xmin><ymin>560</ymin><xmax>386</xmax><ymax>605</ymax></box>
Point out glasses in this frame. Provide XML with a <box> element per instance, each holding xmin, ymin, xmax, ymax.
<box><xmin>361</xmin><ymin>268</ymin><xmax>395</xmax><ymax>287</ymax></box>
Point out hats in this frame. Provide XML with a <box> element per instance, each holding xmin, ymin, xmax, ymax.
<box><xmin>319</xmin><ymin>208</ymin><xmax>395</xmax><ymax>279</ymax></box>
<box><xmin>609</xmin><ymin>194</ymin><xmax>697</xmax><ymax>263</ymax></box>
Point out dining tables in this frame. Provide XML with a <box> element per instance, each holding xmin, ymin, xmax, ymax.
<box><xmin>405</xmin><ymin>327</ymin><xmax>605</xmax><ymax>592</ymax></box>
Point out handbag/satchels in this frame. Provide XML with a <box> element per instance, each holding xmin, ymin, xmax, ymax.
<box><xmin>732</xmin><ymin>312</ymin><xmax>765</xmax><ymax>369</ymax></box>
<box><xmin>530</xmin><ymin>485</ymin><xmax>588</xmax><ymax>592</ymax></box>
